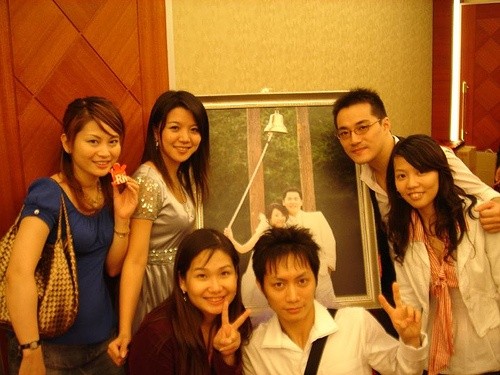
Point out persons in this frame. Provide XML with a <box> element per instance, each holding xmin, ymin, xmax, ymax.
<box><xmin>126</xmin><ymin>227</ymin><xmax>254</xmax><ymax>375</ymax></box>
<box><xmin>488</xmin><ymin>149</ymin><xmax>500</xmax><ymax>194</ymax></box>
<box><xmin>332</xmin><ymin>88</ymin><xmax>500</xmax><ymax>266</ymax></box>
<box><xmin>239</xmin><ymin>224</ymin><xmax>430</xmax><ymax>375</ymax></box>
<box><xmin>225</xmin><ymin>188</ymin><xmax>337</xmax><ymax>307</ymax></box>
<box><xmin>3</xmin><ymin>95</ymin><xmax>141</xmax><ymax>375</ymax></box>
<box><xmin>105</xmin><ymin>89</ymin><xmax>213</xmax><ymax>367</ymax></box>
<box><xmin>386</xmin><ymin>131</ymin><xmax>500</xmax><ymax>375</ymax></box>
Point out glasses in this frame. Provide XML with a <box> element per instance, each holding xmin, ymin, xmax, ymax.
<box><xmin>334</xmin><ymin>119</ymin><xmax>382</xmax><ymax>140</ymax></box>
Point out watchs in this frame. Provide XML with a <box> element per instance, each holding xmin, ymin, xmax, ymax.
<box><xmin>21</xmin><ymin>338</ymin><xmax>41</xmax><ymax>349</ymax></box>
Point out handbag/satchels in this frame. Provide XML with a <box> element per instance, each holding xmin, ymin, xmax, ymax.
<box><xmin>0</xmin><ymin>179</ymin><xmax>80</xmax><ymax>340</ymax></box>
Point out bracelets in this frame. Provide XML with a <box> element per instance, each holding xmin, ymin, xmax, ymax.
<box><xmin>113</xmin><ymin>228</ymin><xmax>131</xmax><ymax>237</ymax></box>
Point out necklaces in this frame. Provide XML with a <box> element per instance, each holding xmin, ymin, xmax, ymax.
<box><xmin>83</xmin><ymin>182</ymin><xmax>103</xmax><ymax>208</ymax></box>
